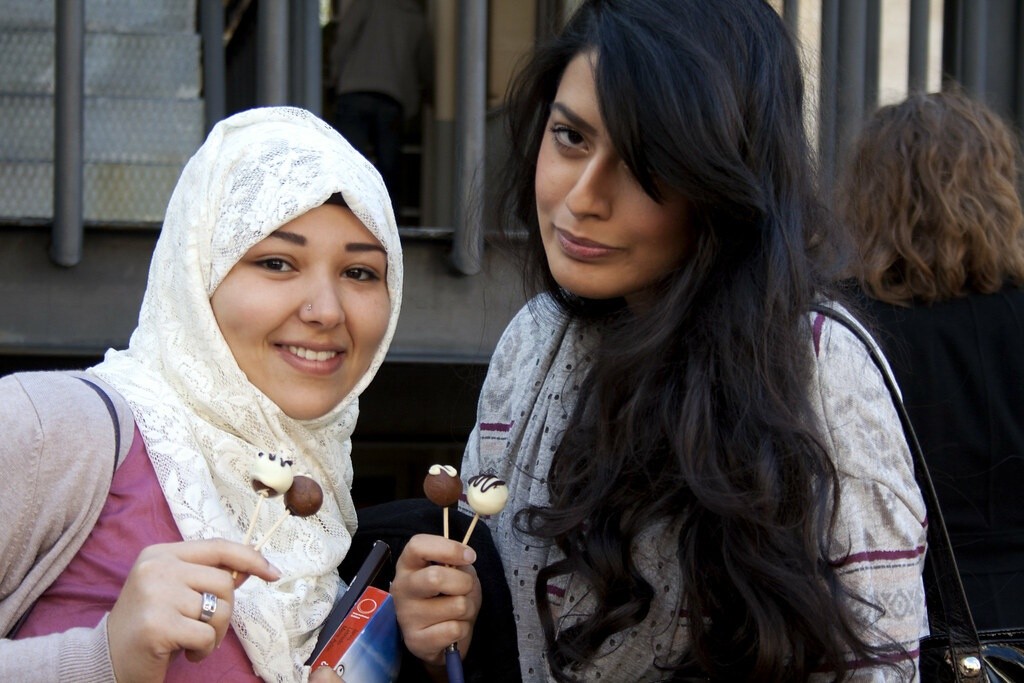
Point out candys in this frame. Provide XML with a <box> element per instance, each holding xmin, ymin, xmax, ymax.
<box><xmin>423</xmin><ymin>465</ymin><xmax>509</xmax><ymax>543</ymax></box>
<box><xmin>233</xmin><ymin>451</ymin><xmax>324</xmax><ymax>579</ymax></box>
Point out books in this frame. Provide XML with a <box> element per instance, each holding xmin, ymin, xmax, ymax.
<box><xmin>303</xmin><ymin>538</ymin><xmax>397</xmax><ymax>667</ymax></box>
<box><xmin>310</xmin><ymin>584</ymin><xmax>399</xmax><ymax>683</ymax></box>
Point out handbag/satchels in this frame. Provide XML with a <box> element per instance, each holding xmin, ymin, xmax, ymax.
<box><xmin>918</xmin><ymin>622</ymin><xmax>1022</xmax><ymax>683</ymax></box>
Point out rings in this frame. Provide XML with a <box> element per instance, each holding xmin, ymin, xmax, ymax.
<box><xmin>199</xmin><ymin>591</ymin><xmax>217</xmax><ymax>623</ymax></box>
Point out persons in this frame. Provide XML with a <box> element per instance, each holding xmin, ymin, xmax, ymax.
<box><xmin>836</xmin><ymin>86</ymin><xmax>1024</xmax><ymax>682</ymax></box>
<box><xmin>387</xmin><ymin>0</ymin><xmax>934</xmax><ymax>682</ymax></box>
<box><xmin>223</xmin><ymin>0</ymin><xmax>438</xmax><ymax>224</ymax></box>
<box><xmin>0</xmin><ymin>103</ymin><xmax>404</xmax><ymax>683</ymax></box>
